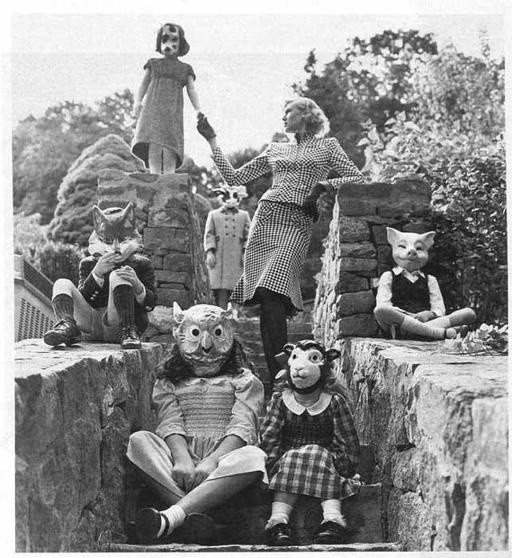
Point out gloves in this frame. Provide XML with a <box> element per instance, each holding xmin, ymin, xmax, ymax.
<box><xmin>197</xmin><ymin>116</ymin><xmax>215</xmax><ymax>141</ymax></box>
<box><xmin>302</xmin><ymin>190</ymin><xmax>320</xmax><ymax>223</ymax></box>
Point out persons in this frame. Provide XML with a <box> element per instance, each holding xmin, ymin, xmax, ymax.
<box><xmin>42</xmin><ymin>202</ymin><xmax>159</xmax><ymax>349</ymax></box>
<box><xmin>130</xmin><ymin>21</ymin><xmax>205</xmax><ymax>174</ymax></box>
<box><xmin>257</xmin><ymin>339</ymin><xmax>362</xmax><ymax>544</ymax></box>
<box><xmin>202</xmin><ymin>184</ymin><xmax>252</xmax><ymax>310</ymax></box>
<box><xmin>373</xmin><ymin>225</ymin><xmax>478</xmax><ymax>340</ymax></box>
<box><xmin>197</xmin><ymin>96</ymin><xmax>364</xmax><ymax>381</ymax></box>
<box><xmin>125</xmin><ymin>301</ymin><xmax>271</xmax><ymax>543</ymax></box>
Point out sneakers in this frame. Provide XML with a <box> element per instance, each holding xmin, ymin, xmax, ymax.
<box><xmin>386</xmin><ymin>322</ymin><xmax>403</xmax><ymax>340</ymax></box>
<box><xmin>183</xmin><ymin>511</ymin><xmax>232</xmax><ymax>544</ymax></box>
<box><xmin>119</xmin><ymin>324</ymin><xmax>141</xmax><ymax>349</ymax></box>
<box><xmin>443</xmin><ymin>324</ymin><xmax>468</xmax><ymax>339</ymax></box>
<box><xmin>42</xmin><ymin>320</ymin><xmax>81</xmax><ymax>345</ymax></box>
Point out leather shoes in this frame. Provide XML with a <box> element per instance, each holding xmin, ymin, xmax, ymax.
<box><xmin>126</xmin><ymin>507</ymin><xmax>170</xmax><ymax>545</ymax></box>
<box><xmin>313</xmin><ymin>521</ymin><xmax>350</xmax><ymax>544</ymax></box>
<box><xmin>266</xmin><ymin>521</ymin><xmax>294</xmax><ymax>545</ymax></box>
